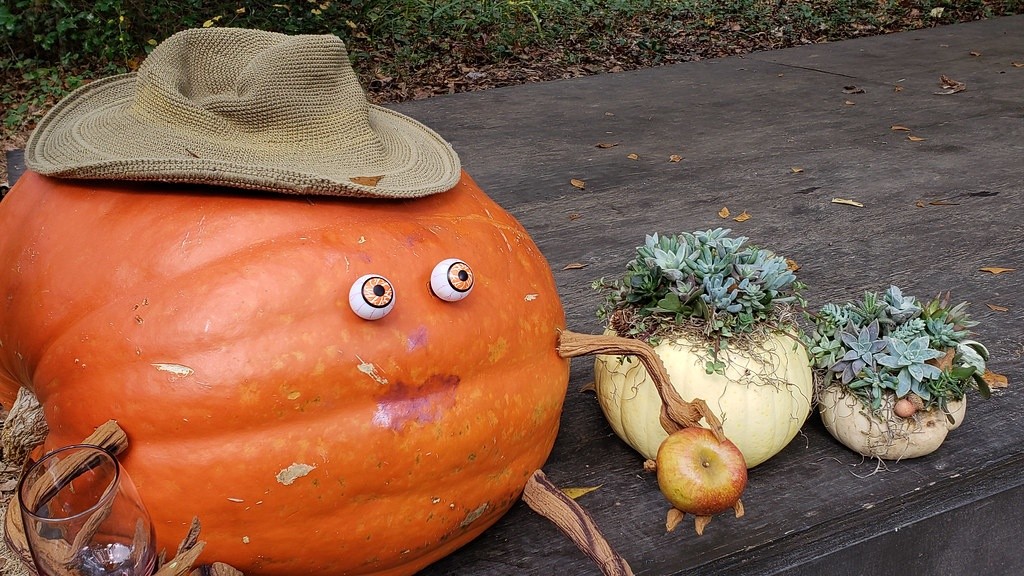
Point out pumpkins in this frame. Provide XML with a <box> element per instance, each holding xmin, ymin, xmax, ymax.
<box><xmin>594</xmin><ymin>309</ymin><xmax>812</xmax><ymax>471</ymax></box>
<box><xmin>819</xmin><ymin>382</ymin><xmax>966</xmax><ymax>460</ymax></box>
<box><xmin>0</xmin><ymin>161</ymin><xmax>573</xmax><ymax>576</ymax></box>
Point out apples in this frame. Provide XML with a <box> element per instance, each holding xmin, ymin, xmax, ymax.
<box><xmin>657</xmin><ymin>427</ymin><xmax>748</xmax><ymax>517</ymax></box>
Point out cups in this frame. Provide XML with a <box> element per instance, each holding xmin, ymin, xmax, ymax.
<box><xmin>18</xmin><ymin>444</ymin><xmax>155</xmax><ymax>576</ymax></box>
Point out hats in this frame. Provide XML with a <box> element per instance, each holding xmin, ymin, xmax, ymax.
<box><xmin>22</xmin><ymin>25</ymin><xmax>464</xmax><ymax>205</ymax></box>
